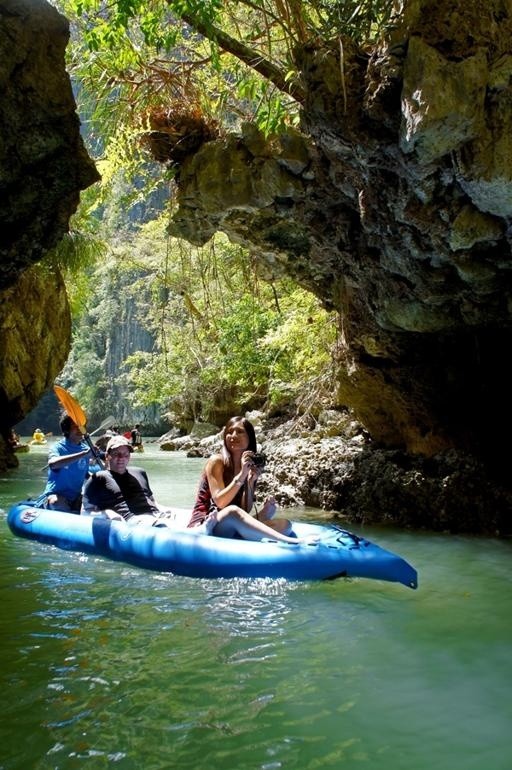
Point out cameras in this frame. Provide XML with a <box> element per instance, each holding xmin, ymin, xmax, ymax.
<box><xmin>250</xmin><ymin>453</ymin><xmax>267</xmax><ymax>467</ymax></box>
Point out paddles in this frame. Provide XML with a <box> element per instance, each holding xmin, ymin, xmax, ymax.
<box><xmin>53</xmin><ymin>384</ymin><xmax>106</xmax><ymax>471</ymax></box>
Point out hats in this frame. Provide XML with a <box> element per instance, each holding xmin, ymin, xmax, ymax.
<box><xmin>106</xmin><ymin>436</ymin><xmax>134</xmax><ymax>453</ymax></box>
<box><xmin>104</xmin><ymin>430</ymin><xmax>113</xmax><ymax>438</ymax></box>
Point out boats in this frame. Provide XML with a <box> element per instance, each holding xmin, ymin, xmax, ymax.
<box><xmin>32</xmin><ymin>439</ymin><xmax>47</xmax><ymax>445</ymax></box>
<box><xmin>7</xmin><ymin>500</ymin><xmax>417</xmax><ymax>590</ymax></box>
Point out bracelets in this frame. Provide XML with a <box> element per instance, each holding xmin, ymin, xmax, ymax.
<box><xmin>233</xmin><ymin>475</ymin><xmax>245</xmax><ymax>487</ymax></box>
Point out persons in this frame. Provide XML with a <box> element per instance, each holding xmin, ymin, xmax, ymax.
<box><xmin>35</xmin><ymin>415</ymin><xmax>106</xmax><ymax>516</ymax></box>
<box><xmin>8</xmin><ymin>424</ymin><xmax>143</xmax><ymax>451</ymax></box>
<box><xmin>186</xmin><ymin>415</ymin><xmax>320</xmax><ymax>545</ymax></box>
<box><xmin>79</xmin><ymin>435</ymin><xmax>218</xmax><ymax>537</ymax></box>
